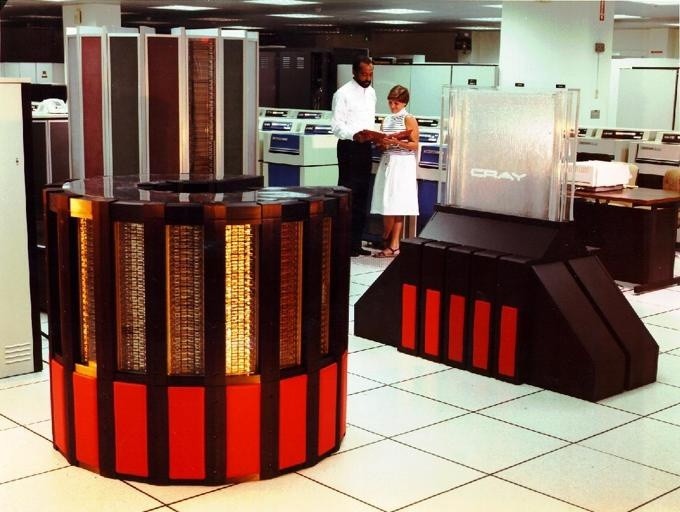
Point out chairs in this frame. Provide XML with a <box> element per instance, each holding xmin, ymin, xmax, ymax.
<box><xmin>625</xmin><ymin>163</ymin><xmax>680</xmax><ymax>220</ymax></box>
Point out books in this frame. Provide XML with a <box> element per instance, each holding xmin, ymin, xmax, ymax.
<box><xmin>361</xmin><ymin>129</ymin><xmax>414</xmax><ymax>144</ymax></box>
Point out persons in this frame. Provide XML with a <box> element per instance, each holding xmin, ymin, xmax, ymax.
<box><xmin>371</xmin><ymin>85</ymin><xmax>420</xmax><ymax>259</ymax></box>
<box><xmin>331</xmin><ymin>57</ymin><xmax>378</xmax><ymax>256</ymax></box>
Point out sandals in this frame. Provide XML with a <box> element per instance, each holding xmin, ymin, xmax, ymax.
<box><xmin>365</xmin><ymin>238</ymin><xmax>401</xmax><ymax>258</ymax></box>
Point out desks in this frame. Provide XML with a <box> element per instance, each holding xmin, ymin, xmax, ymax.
<box><xmin>573</xmin><ymin>185</ymin><xmax>680</xmax><ymax>294</ymax></box>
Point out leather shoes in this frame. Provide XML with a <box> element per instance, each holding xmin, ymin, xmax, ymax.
<box><xmin>352</xmin><ymin>246</ymin><xmax>371</xmax><ymax>257</ymax></box>
